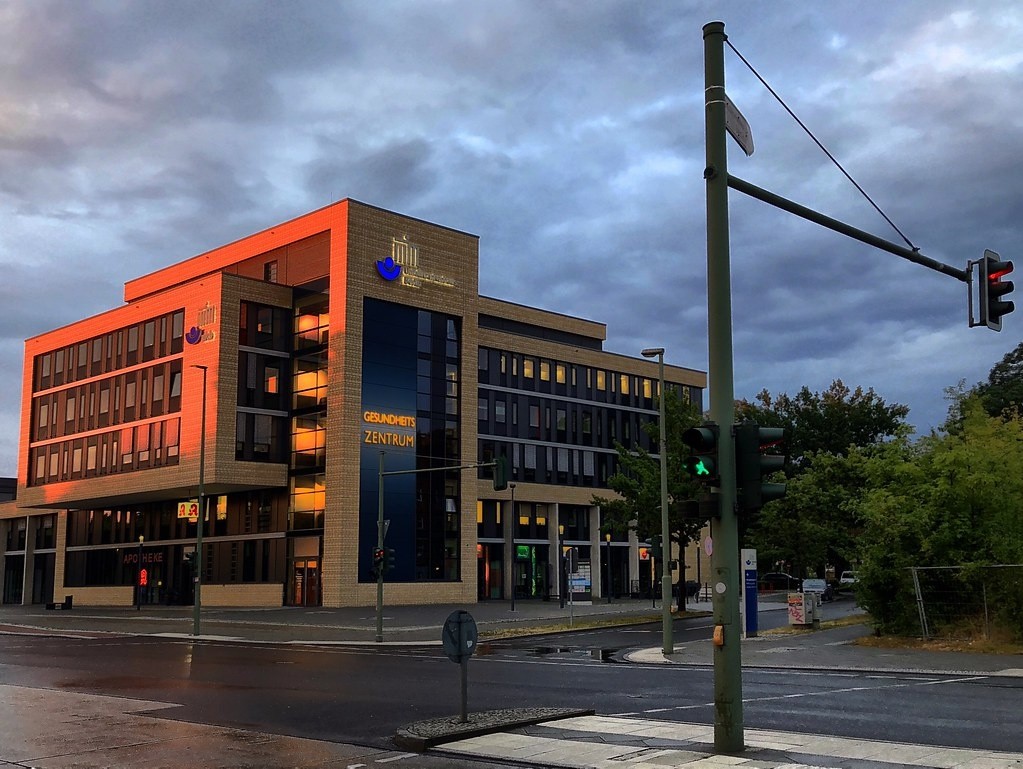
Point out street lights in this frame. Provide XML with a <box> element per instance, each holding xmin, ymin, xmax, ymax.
<box><xmin>190</xmin><ymin>365</ymin><xmax>209</xmax><ymax>636</ymax></box>
<box><xmin>641</xmin><ymin>348</ymin><xmax>673</xmax><ymax>654</ymax></box>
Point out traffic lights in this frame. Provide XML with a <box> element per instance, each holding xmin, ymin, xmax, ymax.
<box><xmin>645</xmin><ymin>535</ymin><xmax>663</xmax><ymax>559</ymax></box>
<box><xmin>372</xmin><ymin>547</ymin><xmax>384</xmax><ymax>567</ymax></box>
<box><xmin>980</xmin><ymin>249</ymin><xmax>1015</xmax><ymax>331</ymax></box>
<box><xmin>681</xmin><ymin>421</ymin><xmax>720</xmax><ymax>482</ymax></box>
<box><xmin>734</xmin><ymin>420</ymin><xmax>787</xmax><ymax>513</ymax></box>
<box><xmin>384</xmin><ymin>547</ymin><xmax>395</xmax><ymax>571</ymax></box>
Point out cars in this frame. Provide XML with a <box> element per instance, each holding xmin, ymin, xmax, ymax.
<box><xmin>840</xmin><ymin>571</ymin><xmax>860</xmax><ymax>583</ymax></box>
<box><xmin>797</xmin><ymin>579</ymin><xmax>833</xmax><ymax>603</ymax></box>
<box><xmin>758</xmin><ymin>573</ymin><xmax>800</xmax><ymax>590</ymax></box>
